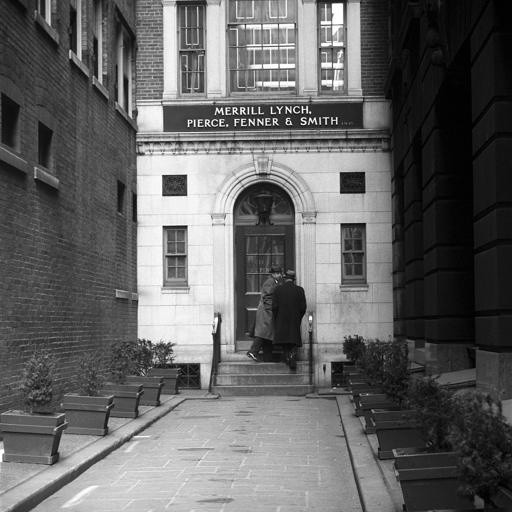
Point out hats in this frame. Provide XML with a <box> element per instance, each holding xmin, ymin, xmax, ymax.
<box><xmin>269</xmin><ymin>268</ymin><xmax>282</xmax><ymax>272</ymax></box>
<box><xmin>283</xmin><ymin>270</ymin><xmax>296</xmax><ymax>281</ymax></box>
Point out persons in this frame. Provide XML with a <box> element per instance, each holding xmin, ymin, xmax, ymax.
<box><xmin>247</xmin><ymin>266</ymin><xmax>283</xmax><ymax>362</ymax></box>
<box><xmin>272</xmin><ymin>270</ymin><xmax>306</xmax><ymax>368</ymax></box>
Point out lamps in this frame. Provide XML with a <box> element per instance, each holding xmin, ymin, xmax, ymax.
<box><xmin>254</xmin><ymin>193</ymin><xmax>274</xmax><ymax>226</ymax></box>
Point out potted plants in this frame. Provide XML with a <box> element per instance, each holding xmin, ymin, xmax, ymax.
<box><xmin>0</xmin><ymin>338</ymin><xmax>184</xmax><ymax>465</ymax></box>
<box><xmin>342</xmin><ymin>333</ymin><xmax>512</xmax><ymax>511</ymax></box>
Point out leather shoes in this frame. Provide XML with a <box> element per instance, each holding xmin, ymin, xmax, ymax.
<box><xmin>247</xmin><ymin>351</ymin><xmax>257</xmax><ymax>360</ymax></box>
<box><xmin>286</xmin><ymin>358</ymin><xmax>296</xmax><ymax>367</ymax></box>
<box><xmin>264</xmin><ymin>357</ymin><xmax>280</xmax><ymax>362</ymax></box>
